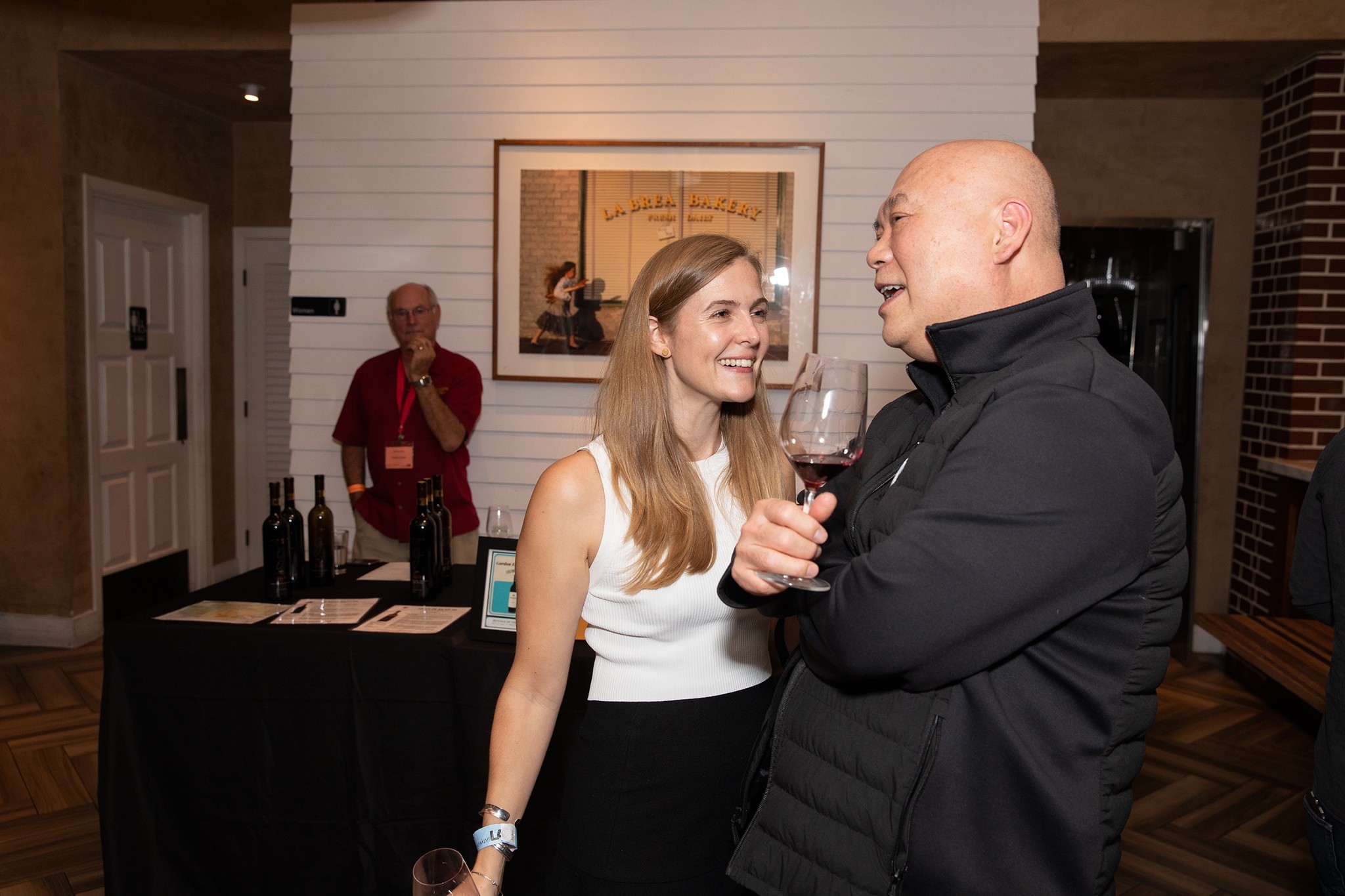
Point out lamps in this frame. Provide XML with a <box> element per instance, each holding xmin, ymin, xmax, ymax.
<box><xmin>244</xmin><ymin>84</ymin><xmax>260</xmax><ymax>102</ymax></box>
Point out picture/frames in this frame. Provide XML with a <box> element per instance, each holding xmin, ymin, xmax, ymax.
<box><xmin>468</xmin><ymin>534</ymin><xmax>522</xmax><ymax>644</ymax></box>
<box><xmin>488</xmin><ymin>139</ymin><xmax>826</xmax><ymax>393</ymax></box>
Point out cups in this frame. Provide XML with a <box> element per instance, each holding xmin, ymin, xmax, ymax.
<box><xmin>332</xmin><ymin>528</ymin><xmax>348</xmax><ymax>575</ymax></box>
<box><xmin>487</xmin><ymin>505</ymin><xmax>512</xmax><ymax>538</ymax></box>
<box><xmin>413</xmin><ymin>847</ymin><xmax>480</xmax><ymax>896</ymax></box>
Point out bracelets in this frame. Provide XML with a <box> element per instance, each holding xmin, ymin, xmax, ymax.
<box><xmin>347</xmin><ymin>483</ymin><xmax>365</xmax><ymax>494</ymax></box>
<box><xmin>472</xmin><ymin>803</ymin><xmax>522</xmax><ymax>862</ymax></box>
<box><xmin>471</xmin><ymin>872</ymin><xmax>504</xmax><ymax>896</ymax></box>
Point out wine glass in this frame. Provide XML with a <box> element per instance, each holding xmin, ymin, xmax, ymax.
<box><xmin>755</xmin><ymin>352</ymin><xmax>871</xmax><ymax>592</ymax></box>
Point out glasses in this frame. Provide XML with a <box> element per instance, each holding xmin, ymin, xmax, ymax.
<box><xmin>389</xmin><ymin>305</ymin><xmax>436</xmax><ymax>320</ymax></box>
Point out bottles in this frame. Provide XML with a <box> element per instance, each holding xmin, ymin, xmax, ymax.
<box><xmin>292</xmin><ymin>604</ymin><xmax>307</xmax><ymax>613</ymax></box>
<box><xmin>508</xmin><ymin>575</ymin><xmax>517</xmax><ymax>613</ymax></box>
<box><xmin>378</xmin><ymin>610</ymin><xmax>402</xmax><ymax>622</ymax></box>
<box><xmin>264</xmin><ymin>473</ymin><xmax>457</xmax><ymax>603</ymax></box>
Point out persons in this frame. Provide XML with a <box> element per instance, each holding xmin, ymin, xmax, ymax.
<box><xmin>721</xmin><ymin>137</ymin><xmax>1189</xmax><ymax>895</ymax></box>
<box><xmin>331</xmin><ymin>282</ymin><xmax>483</xmax><ymax>564</ymax></box>
<box><xmin>1290</xmin><ymin>426</ymin><xmax>1345</xmax><ymax>896</ymax></box>
<box><xmin>530</xmin><ymin>261</ymin><xmax>590</xmax><ymax>350</ymax></box>
<box><xmin>450</xmin><ymin>234</ymin><xmax>797</xmax><ymax>896</ymax></box>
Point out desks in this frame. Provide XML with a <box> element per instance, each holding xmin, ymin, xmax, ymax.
<box><xmin>103</xmin><ymin>565</ymin><xmax>599</xmax><ymax>896</ymax></box>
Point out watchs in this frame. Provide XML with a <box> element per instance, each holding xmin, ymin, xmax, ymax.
<box><xmin>411</xmin><ymin>374</ymin><xmax>432</xmax><ymax>387</ymax></box>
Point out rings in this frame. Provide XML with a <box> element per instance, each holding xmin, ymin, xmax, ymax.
<box><xmin>418</xmin><ymin>346</ymin><xmax>424</xmax><ymax>351</ymax></box>
